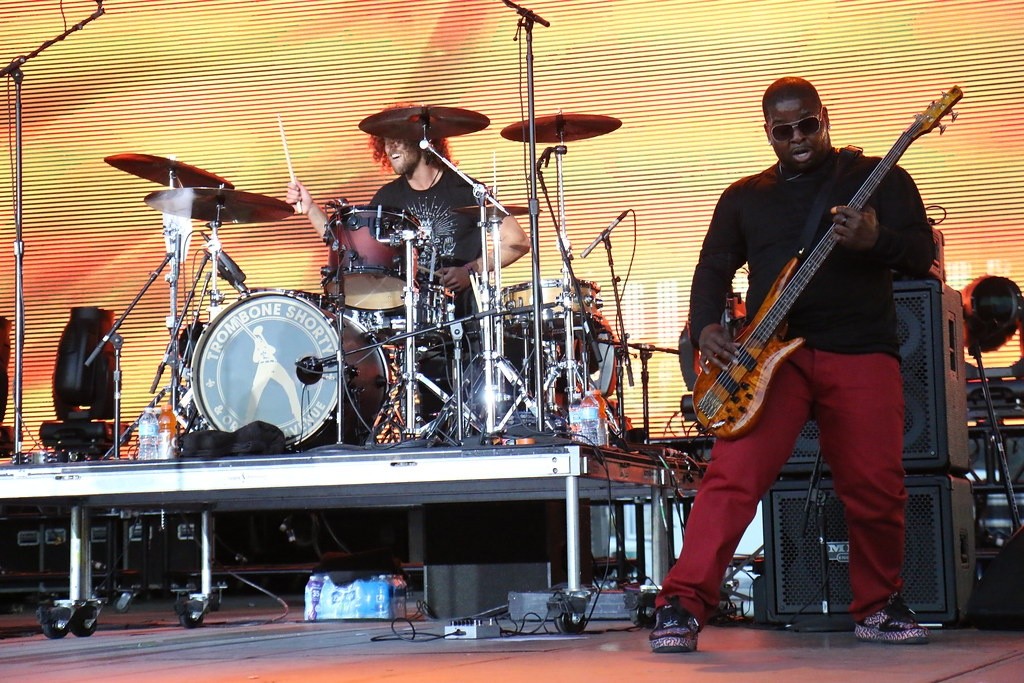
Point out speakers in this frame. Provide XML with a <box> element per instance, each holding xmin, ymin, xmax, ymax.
<box><xmin>777</xmin><ymin>279</ymin><xmax>971</xmax><ymax>475</ymax></box>
<box><xmin>762</xmin><ymin>474</ymin><xmax>975</xmax><ymax>624</ymax></box>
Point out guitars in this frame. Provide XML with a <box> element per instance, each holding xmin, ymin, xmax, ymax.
<box><xmin>691</xmin><ymin>83</ymin><xmax>966</xmax><ymax>442</ymax></box>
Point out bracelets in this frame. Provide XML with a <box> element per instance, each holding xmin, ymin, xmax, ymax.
<box><xmin>464</xmin><ymin>263</ymin><xmax>477</xmax><ymax>277</ymax></box>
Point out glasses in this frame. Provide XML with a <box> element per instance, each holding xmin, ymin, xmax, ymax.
<box><xmin>767</xmin><ymin>108</ymin><xmax>822</xmax><ymax>143</ymax></box>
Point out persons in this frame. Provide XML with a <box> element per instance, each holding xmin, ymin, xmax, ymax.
<box><xmin>649</xmin><ymin>76</ymin><xmax>935</xmax><ymax>652</ymax></box>
<box><xmin>286</xmin><ymin>102</ymin><xmax>529</xmax><ymax>327</ymax></box>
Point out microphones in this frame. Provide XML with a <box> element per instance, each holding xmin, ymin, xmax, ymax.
<box><xmin>580</xmin><ymin>211</ymin><xmax>629</xmax><ymax>258</ymax></box>
<box><xmin>201</xmin><ymin>232</ymin><xmax>246</xmax><ymax>283</ymax></box>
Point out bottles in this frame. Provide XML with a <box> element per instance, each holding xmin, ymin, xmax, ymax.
<box><xmin>304</xmin><ymin>573</ymin><xmax>407</xmax><ymax>622</ymax></box>
<box><xmin>139</xmin><ymin>405</ymin><xmax>178</xmax><ymax>462</ymax></box>
<box><xmin>571</xmin><ymin>389</ymin><xmax>608</xmax><ymax>447</ymax></box>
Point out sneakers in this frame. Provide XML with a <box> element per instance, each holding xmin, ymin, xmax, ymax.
<box><xmin>648</xmin><ymin>603</ymin><xmax>700</xmax><ymax>651</ymax></box>
<box><xmin>854</xmin><ymin>592</ymin><xmax>929</xmax><ymax>644</ymax></box>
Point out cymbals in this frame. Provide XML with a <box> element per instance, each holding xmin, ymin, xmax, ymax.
<box><xmin>357</xmin><ymin>104</ymin><xmax>490</xmax><ymax>139</ymax></box>
<box><xmin>500</xmin><ymin>107</ymin><xmax>622</xmax><ymax>143</ymax></box>
<box><xmin>103</xmin><ymin>153</ymin><xmax>235</xmax><ymax>189</ymax></box>
<box><xmin>451</xmin><ymin>203</ymin><xmax>543</xmax><ymax>218</ymax></box>
<box><xmin>144</xmin><ymin>182</ymin><xmax>295</xmax><ymax>224</ymax></box>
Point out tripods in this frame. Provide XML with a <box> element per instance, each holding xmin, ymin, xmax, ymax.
<box><xmin>362</xmin><ymin>140</ymin><xmax>622</xmax><ymax>450</ymax></box>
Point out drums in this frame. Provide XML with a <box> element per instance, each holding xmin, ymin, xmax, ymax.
<box><xmin>501</xmin><ymin>278</ymin><xmax>604</xmax><ymax>341</ymax></box>
<box><xmin>320</xmin><ymin>204</ymin><xmax>425</xmax><ymax>313</ymax></box>
<box><xmin>189</xmin><ymin>286</ymin><xmax>390</xmax><ymax>454</ymax></box>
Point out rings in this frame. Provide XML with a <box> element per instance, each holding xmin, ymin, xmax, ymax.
<box><xmin>841</xmin><ymin>217</ymin><xmax>848</xmax><ymax>225</ymax></box>
